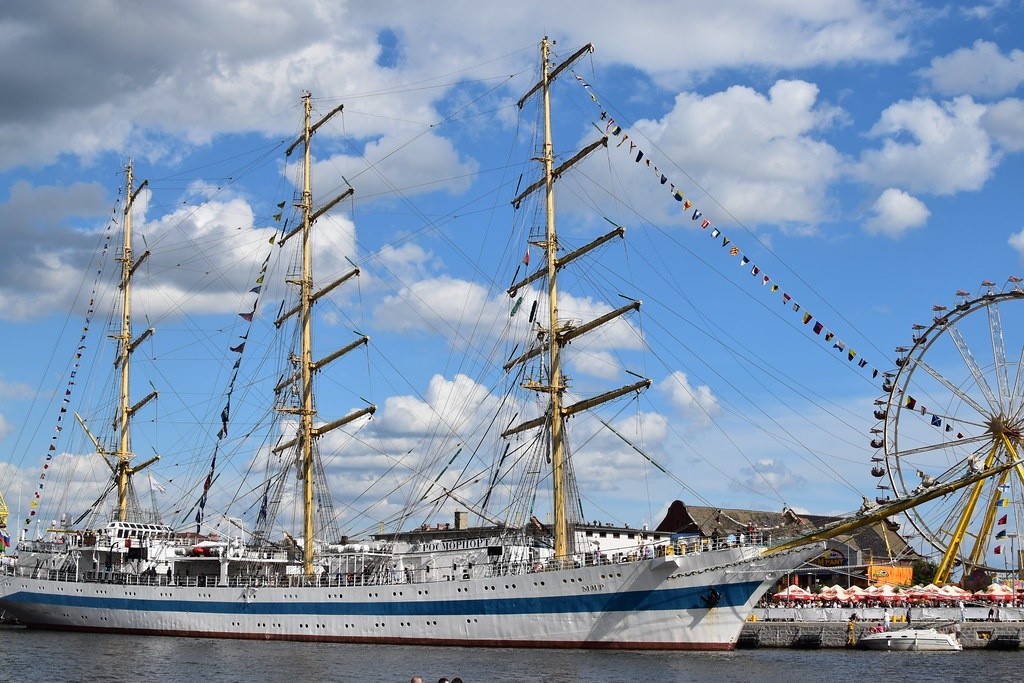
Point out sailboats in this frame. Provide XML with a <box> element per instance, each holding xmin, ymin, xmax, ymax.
<box><xmin>0</xmin><ymin>36</ymin><xmax>1000</xmax><ymax>650</ymax></box>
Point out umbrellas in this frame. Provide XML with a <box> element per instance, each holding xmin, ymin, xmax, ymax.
<box><xmin>774</xmin><ymin>584</ymin><xmax>1023</xmax><ymax>605</ymax></box>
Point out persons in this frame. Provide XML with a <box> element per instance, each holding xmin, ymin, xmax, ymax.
<box><xmin>590</xmin><ymin>522</ymin><xmax>759</xmax><ymax>563</ymax></box>
<box><xmin>138</xmin><ymin>565</ymin><xmax>205</xmax><ymax>587</ymax></box>
<box><xmin>55</xmin><ymin>528</ymin><xmax>107</xmax><ymax>547</ymax></box>
<box><xmin>413</xmin><ymin>675</ymin><xmax>466</xmax><ymax>683</ymax></box>
<box><xmin>874</xmin><ymin>623</ymin><xmax>882</xmax><ymax>633</ymax></box>
<box><xmin>759</xmin><ymin>597</ymin><xmax>1024</xmax><ymax>621</ymax></box>
<box><xmin>905</xmin><ymin>608</ymin><xmax>912</xmax><ymax>629</ymax></box>
<box><xmin>845</xmin><ymin>617</ymin><xmax>856</xmax><ymax>645</ymax></box>
<box><xmin>881</xmin><ymin>608</ymin><xmax>890</xmax><ymax>631</ymax></box>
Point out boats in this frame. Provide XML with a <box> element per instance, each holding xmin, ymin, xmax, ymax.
<box><xmin>860</xmin><ymin>628</ymin><xmax>962</xmax><ymax>651</ymax></box>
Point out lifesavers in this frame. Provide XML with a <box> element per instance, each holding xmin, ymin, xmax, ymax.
<box><xmin>869</xmin><ymin>627</ymin><xmax>878</xmax><ymax>634</ymax></box>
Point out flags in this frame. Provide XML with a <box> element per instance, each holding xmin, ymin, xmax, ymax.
<box><xmin>573</xmin><ymin>73</ymin><xmax>1009</xmax><ymax>554</ymax></box>
